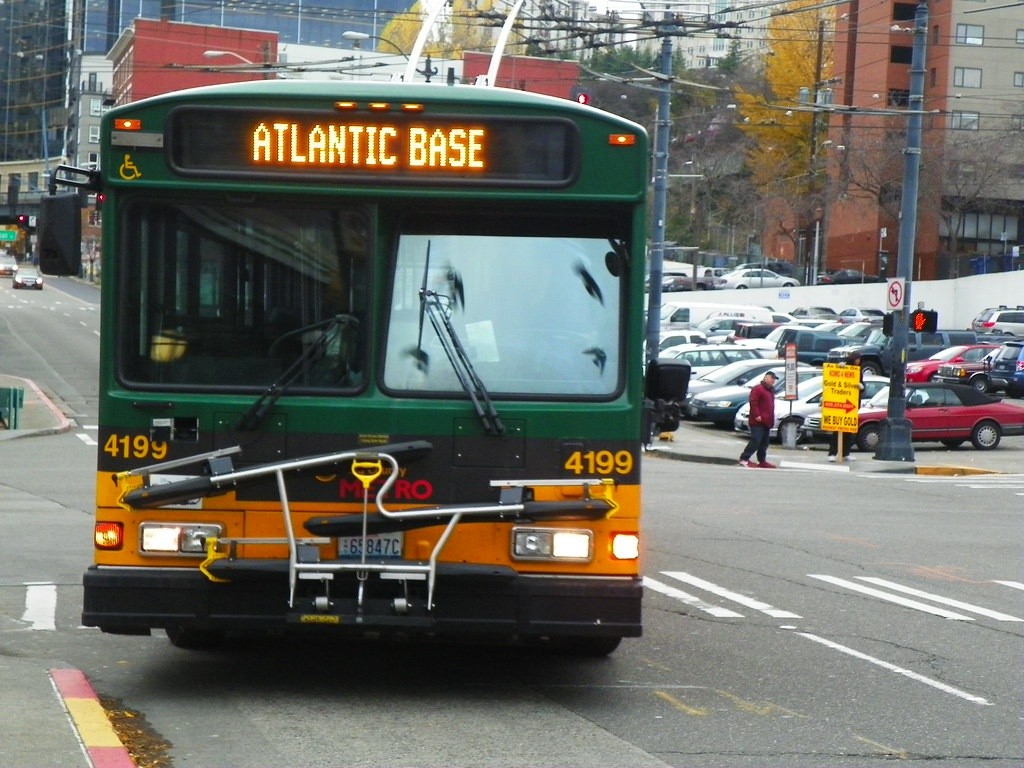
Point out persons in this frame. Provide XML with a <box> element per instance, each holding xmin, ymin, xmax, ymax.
<box><xmin>827</xmin><ymin>352</ymin><xmax>865</xmax><ymax>462</ymax></box>
<box><xmin>739</xmin><ymin>371</ymin><xmax>780</xmax><ymax>468</ymax></box>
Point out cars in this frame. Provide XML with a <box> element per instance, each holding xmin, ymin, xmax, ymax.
<box><xmin>0</xmin><ymin>249</ymin><xmax>42</xmax><ymax>290</ymax></box>
<box><xmin>972</xmin><ymin>305</ymin><xmax>1024</xmax><ymax>338</ymax></box>
<box><xmin>733</xmin><ymin>375</ymin><xmax>929</xmax><ymax>445</ymax></box>
<box><xmin>645</xmin><ymin>302</ymin><xmax>884</xmax><ymax>367</ymax></box>
<box><xmin>906</xmin><ymin>344</ymin><xmax>1000</xmax><ymax>383</ymax></box>
<box><xmin>932</xmin><ymin>349</ymin><xmax>996</xmax><ymax>394</ymax></box>
<box><xmin>642</xmin><ymin>330</ymin><xmax>765</xmax><ymax>380</ymax></box>
<box><xmin>989</xmin><ymin>341</ymin><xmax>1024</xmax><ymax>399</ymax></box>
<box><xmin>816</xmin><ymin>269</ymin><xmax>888</xmax><ymax>285</ymax></box>
<box><xmin>799</xmin><ymin>383</ymin><xmax>1024</xmax><ymax>451</ymax></box>
<box><xmin>713</xmin><ymin>261</ymin><xmax>801</xmax><ymax>289</ymax></box>
<box><xmin>826</xmin><ymin>328</ymin><xmax>977</xmax><ymax>377</ymax></box>
<box><xmin>682</xmin><ymin>359</ymin><xmax>813</xmax><ymax>420</ymax></box>
<box><xmin>644</xmin><ymin>268</ymin><xmax>733</xmax><ymax>292</ymax></box>
<box><xmin>687</xmin><ymin>367</ymin><xmax>824</xmax><ymax>430</ymax></box>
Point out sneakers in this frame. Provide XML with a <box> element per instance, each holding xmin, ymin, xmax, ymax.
<box><xmin>739</xmin><ymin>459</ymin><xmax>758</xmax><ymax>467</ymax></box>
<box><xmin>759</xmin><ymin>461</ymin><xmax>776</xmax><ymax>468</ymax></box>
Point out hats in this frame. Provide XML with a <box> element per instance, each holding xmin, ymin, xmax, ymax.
<box><xmin>766</xmin><ymin>371</ymin><xmax>779</xmax><ymax>380</ymax></box>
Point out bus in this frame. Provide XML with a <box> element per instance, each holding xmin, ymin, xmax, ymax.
<box><xmin>39</xmin><ymin>79</ymin><xmax>692</xmax><ymax>659</ymax></box>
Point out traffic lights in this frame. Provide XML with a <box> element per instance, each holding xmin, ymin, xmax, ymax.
<box><xmin>909</xmin><ymin>309</ymin><xmax>937</xmax><ymax>332</ymax></box>
<box><xmin>572</xmin><ymin>87</ymin><xmax>592</xmax><ymax>105</ymax></box>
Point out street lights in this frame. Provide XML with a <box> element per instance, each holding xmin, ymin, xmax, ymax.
<box><xmin>202</xmin><ymin>31</ymin><xmax>437</xmax><ymax>82</ymax></box>
<box><xmin>805</xmin><ymin>140</ymin><xmax>832</xmax><ymax>286</ymax></box>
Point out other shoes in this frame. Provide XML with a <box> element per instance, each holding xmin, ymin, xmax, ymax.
<box><xmin>842</xmin><ymin>455</ymin><xmax>856</xmax><ymax>462</ymax></box>
<box><xmin>828</xmin><ymin>455</ymin><xmax>835</xmax><ymax>462</ymax></box>
